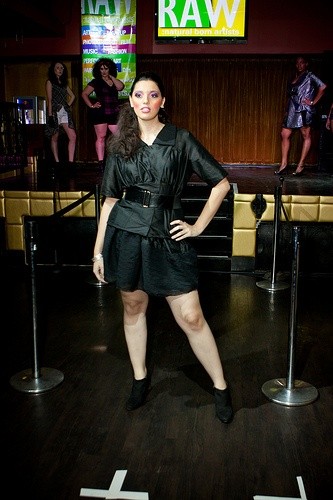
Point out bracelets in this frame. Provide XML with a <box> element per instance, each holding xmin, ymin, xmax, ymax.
<box><xmin>310</xmin><ymin>101</ymin><xmax>314</xmax><ymax>106</ymax></box>
<box><xmin>91</xmin><ymin>104</ymin><xmax>93</xmax><ymax>108</ymax></box>
<box><xmin>91</xmin><ymin>254</ymin><xmax>104</xmax><ymax>263</ymax></box>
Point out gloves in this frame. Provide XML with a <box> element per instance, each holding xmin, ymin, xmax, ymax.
<box><xmin>48</xmin><ymin>116</ymin><xmax>55</xmax><ymax>128</ymax></box>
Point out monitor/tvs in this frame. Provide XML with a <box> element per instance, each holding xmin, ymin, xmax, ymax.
<box><xmin>155</xmin><ymin>0</ymin><xmax>249</xmax><ymax>44</ymax></box>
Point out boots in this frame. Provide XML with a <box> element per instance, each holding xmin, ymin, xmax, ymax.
<box><xmin>54</xmin><ymin>162</ymin><xmax>80</xmax><ymax>177</ymax></box>
<box><xmin>98</xmin><ymin>161</ymin><xmax>105</xmax><ymax>177</ymax></box>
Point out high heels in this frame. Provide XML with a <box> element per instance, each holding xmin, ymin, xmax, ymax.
<box><xmin>212</xmin><ymin>380</ymin><xmax>234</xmax><ymax>424</ymax></box>
<box><xmin>291</xmin><ymin>167</ymin><xmax>305</xmax><ymax>176</ymax></box>
<box><xmin>274</xmin><ymin>166</ymin><xmax>288</xmax><ymax>175</ymax></box>
<box><xmin>126</xmin><ymin>370</ymin><xmax>151</xmax><ymax>411</ymax></box>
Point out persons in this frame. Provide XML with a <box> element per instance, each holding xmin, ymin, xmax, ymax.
<box><xmin>325</xmin><ymin>103</ymin><xmax>333</xmax><ymax>133</ymax></box>
<box><xmin>91</xmin><ymin>74</ymin><xmax>235</xmax><ymax>426</ymax></box>
<box><xmin>81</xmin><ymin>59</ymin><xmax>125</xmax><ymax>176</ymax></box>
<box><xmin>45</xmin><ymin>60</ymin><xmax>79</xmax><ymax>167</ymax></box>
<box><xmin>273</xmin><ymin>55</ymin><xmax>326</xmax><ymax>176</ymax></box>
